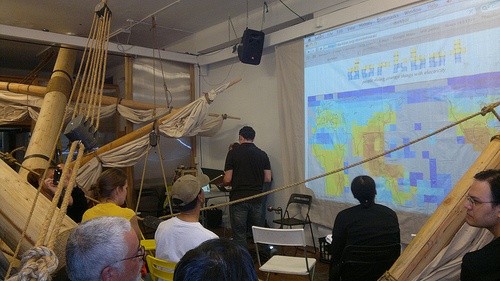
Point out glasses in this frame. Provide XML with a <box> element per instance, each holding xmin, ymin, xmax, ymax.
<box><xmin>464</xmin><ymin>195</ymin><xmax>499</xmax><ymax>208</ymax></box>
<box><xmin>99</xmin><ymin>245</ymin><xmax>146</xmax><ymax>272</ymax></box>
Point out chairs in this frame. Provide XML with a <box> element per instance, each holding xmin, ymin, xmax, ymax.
<box><xmin>273</xmin><ymin>194</ymin><xmax>316</xmax><ymax>256</ymax></box>
<box><xmin>146</xmin><ymin>255</ymin><xmax>177</xmax><ymax>281</ymax></box>
<box><xmin>252</xmin><ymin>225</ymin><xmax>316</xmax><ymax>281</ymax></box>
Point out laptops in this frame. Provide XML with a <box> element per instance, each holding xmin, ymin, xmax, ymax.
<box><xmin>201</xmin><ymin>168</ymin><xmax>225</xmax><ymax>185</ymax></box>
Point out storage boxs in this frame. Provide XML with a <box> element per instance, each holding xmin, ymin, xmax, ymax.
<box><xmin>318</xmin><ymin>237</ymin><xmax>332</xmax><ymax>264</ymax></box>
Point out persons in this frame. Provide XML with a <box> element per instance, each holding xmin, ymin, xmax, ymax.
<box><xmin>460</xmin><ymin>169</ymin><xmax>500</xmax><ymax>281</ymax></box>
<box><xmin>26</xmin><ymin>163</ymin><xmax>87</xmax><ymax>224</ymax></box>
<box><xmin>154</xmin><ymin>174</ymin><xmax>220</xmax><ymax>281</ymax></box>
<box><xmin>223</xmin><ymin>126</ymin><xmax>271</xmax><ymax>266</ymax></box>
<box><xmin>65</xmin><ymin>216</ymin><xmax>146</xmax><ymax>281</ymax></box>
<box><xmin>81</xmin><ymin>169</ymin><xmax>142</xmax><ymax>242</ymax></box>
<box><xmin>173</xmin><ymin>239</ymin><xmax>258</xmax><ymax>281</ymax></box>
<box><xmin>329</xmin><ymin>175</ymin><xmax>401</xmax><ymax>281</ymax></box>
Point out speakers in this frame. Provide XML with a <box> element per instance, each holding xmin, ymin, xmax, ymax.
<box><xmin>237</xmin><ymin>29</ymin><xmax>264</xmax><ymax>65</ymax></box>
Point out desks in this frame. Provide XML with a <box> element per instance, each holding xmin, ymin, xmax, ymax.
<box><xmin>200</xmin><ymin>191</ymin><xmax>231</xmax><ymax>229</ymax></box>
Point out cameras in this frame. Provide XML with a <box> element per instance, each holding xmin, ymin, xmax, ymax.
<box><xmin>53</xmin><ymin>169</ymin><xmax>62</xmax><ymax>185</ymax></box>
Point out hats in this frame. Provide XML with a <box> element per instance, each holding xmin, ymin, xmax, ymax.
<box><xmin>170</xmin><ymin>174</ymin><xmax>210</xmax><ymax>204</ymax></box>
